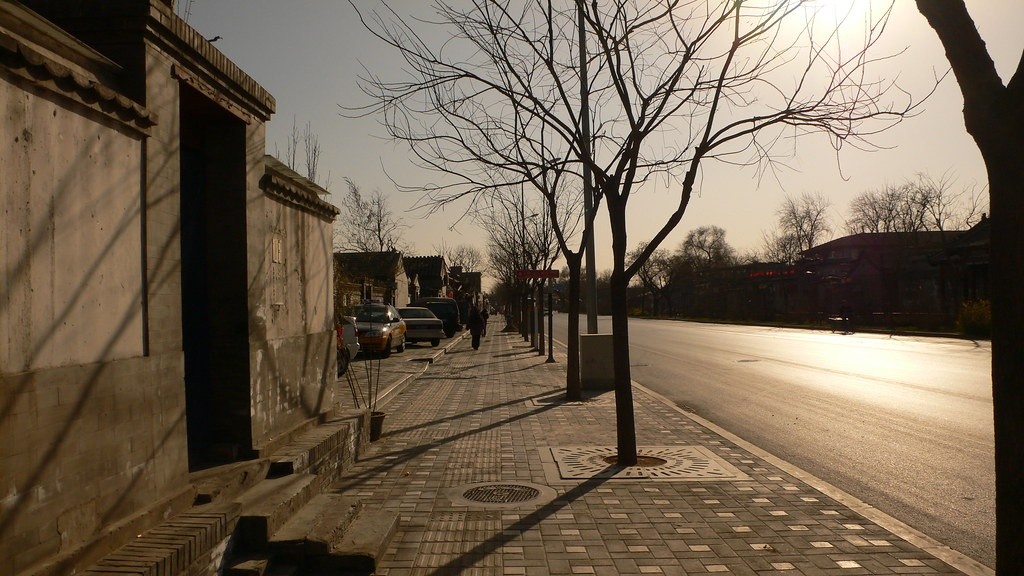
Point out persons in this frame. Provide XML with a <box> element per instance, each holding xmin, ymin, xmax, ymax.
<box><xmin>481</xmin><ymin>309</ymin><xmax>489</xmax><ymax>338</ymax></box>
<box><xmin>466</xmin><ymin>306</ymin><xmax>485</xmax><ymax>350</ymax></box>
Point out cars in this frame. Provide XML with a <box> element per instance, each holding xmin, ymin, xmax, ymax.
<box><xmin>345</xmin><ymin>299</ymin><xmax>407</xmax><ymax>358</ymax></box>
<box><xmin>394</xmin><ymin>306</ymin><xmax>445</xmax><ymax>347</ymax></box>
<box><xmin>337</xmin><ymin>314</ymin><xmax>360</xmax><ymax>380</ymax></box>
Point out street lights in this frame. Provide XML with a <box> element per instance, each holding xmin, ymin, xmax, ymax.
<box><xmin>514</xmin><ymin>213</ymin><xmax>539</xmax><ymax>333</ymax></box>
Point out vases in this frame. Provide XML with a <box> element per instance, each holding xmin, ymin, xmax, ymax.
<box><xmin>368</xmin><ymin>411</ymin><xmax>386</xmax><ymax>440</ymax></box>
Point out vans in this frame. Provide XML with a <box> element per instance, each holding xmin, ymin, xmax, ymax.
<box><xmin>406</xmin><ymin>296</ymin><xmax>460</xmax><ymax>337</ymax></box>
